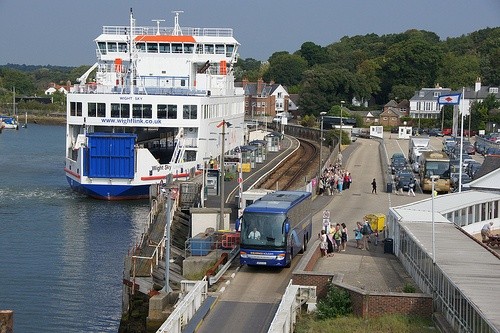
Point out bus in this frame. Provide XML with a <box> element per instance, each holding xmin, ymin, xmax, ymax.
<box><xmin>235</xmin><ymin>189</ymin><xmax>312</xmax><ymax>271</ymax></box>
<box><xmin>413</xmin><ymin>148</ymin><xmax>451</xmax><ymax>194</ymax></box>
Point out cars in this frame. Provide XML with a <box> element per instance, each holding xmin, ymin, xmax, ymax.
<box><xmin>393</xmin><ymin>124</ymin><xmax>473</xmax><ymax>137</ymax></box>
<box><xmin>390</xmin><ymin>151</ymin><xmax>416</xmax><ymax>192</ymax></box>
<box><xmin>444</xmin><ymin>132</ymin><xmax>499</xmax><ymax>188</ymax></box>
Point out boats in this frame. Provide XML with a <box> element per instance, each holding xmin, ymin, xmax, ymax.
<box><xmin>64</xmin><ymin>4</ymin><xmax>246</xmax><ymax>202</ymax></box>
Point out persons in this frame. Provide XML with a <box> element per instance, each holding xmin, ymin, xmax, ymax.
<box><xmin>481</xmin><ymin>222</ymin><xmax>494</xmax><ymax>241</ymax></box>
<box><xmin>371</xmin><ymin>178</ymin><xmax>377</xmax><ymax>195</ymax></box>
<box><xmin>431</xmin><ymin>188</ymin><xmax>438</xmax><ymax>197</ymax></box>
<box><xmin>317</xmin><ymin>151</ymin><xmax>353</xmax><ymax>196</ymax></box>
<box><xmin>318</xmin><ymin>220</ymin><xmax>373</xmax><ymax>259</ymax></box>
<box><xmin>393</xmin><ymin>175</ymin><xmax>417</xmax><ymax>197</ymax></box>
<box><xmin>248</xmin><ymin>227</ymin><xmax>261</xmax><ymax>240</ymax></box>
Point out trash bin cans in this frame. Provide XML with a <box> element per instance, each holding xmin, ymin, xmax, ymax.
<box><xmin>387</xmin><ymin>182</ymin><xmax>392</xmax><ymax>193</ymax></box>
<box><xmin>384</xmin><ymin>238</ymin><xmax>393</xmax><ymax>253</ymax></box>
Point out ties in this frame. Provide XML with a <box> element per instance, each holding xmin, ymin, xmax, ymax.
<box><xmin>254</xmin><ymin>231</ymin><xmax>256</xmax><ymax>239</ymax></box>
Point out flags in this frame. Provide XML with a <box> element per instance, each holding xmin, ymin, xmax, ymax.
<box><xmin>438</xmin><ymin>93</ymin><xmax>462</xmax><ymax>106</ymax></box>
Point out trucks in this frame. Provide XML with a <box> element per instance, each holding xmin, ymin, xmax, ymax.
<box><xmin>409</xmin><ymin>137</ymin><xmax>430</xmax><ymax>160</ymax></box>
